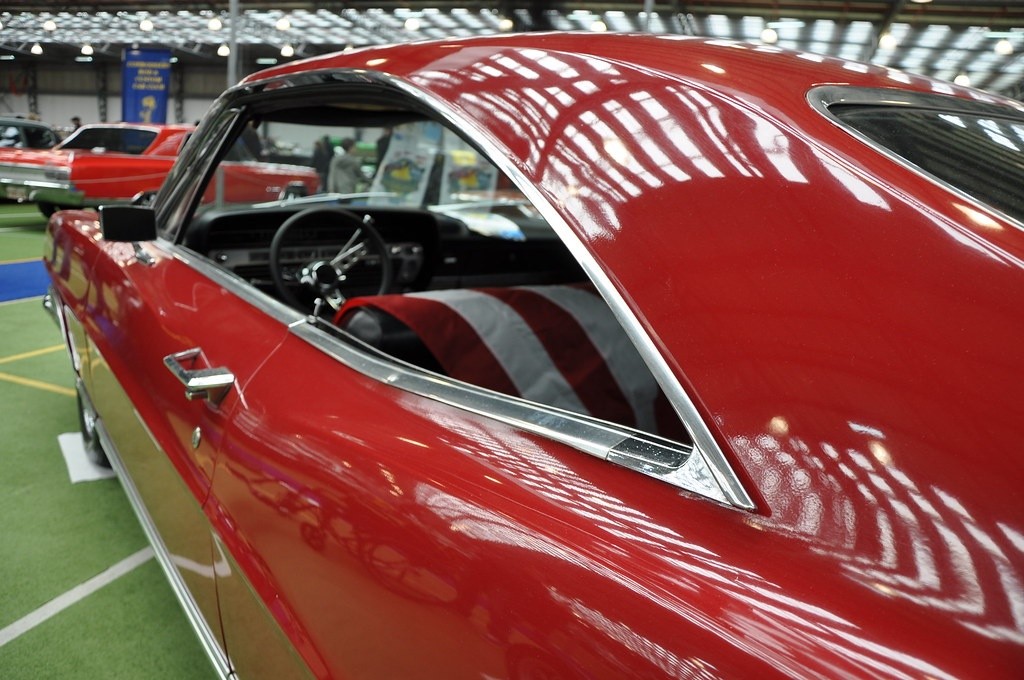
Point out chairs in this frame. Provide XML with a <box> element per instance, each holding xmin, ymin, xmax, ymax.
<box><xmin>332</xmin><ymin>281</ymin><xmax>700</xmax><ymax>450</ymax></box>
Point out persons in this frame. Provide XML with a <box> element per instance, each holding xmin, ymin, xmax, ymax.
<box><xmin>375</xmin><ymin>127</ymin><xmax>393</xmax><ymax>171</ymax></box>
<box><xmin>326</xmin><ymin>138</ymin><xmax>369</xmax><ymax>193</ymax></box>
<box><xmin>314</xmin><ymin>137</ymin><xmax>333</xmax><ymax>174</ymax></box>
<box><xmin>67</xmin><ymin>117</ymin><xmax>81</xmax><ymax>136</ymax></box>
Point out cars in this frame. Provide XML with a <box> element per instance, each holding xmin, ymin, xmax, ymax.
<box><xmin>0</xmin><ymin>117</ymin><xmax>64</xmax><ymax>202</ymax></box>
<box><xmin>34</xmin><ymin>32</ymin><xmax>1024</xmax><ymax>679</ymax></box>
<box><xmin>0</xmin><ymin>121</ymin><xmax>322</xmax><ymax>230</ymax></box>
